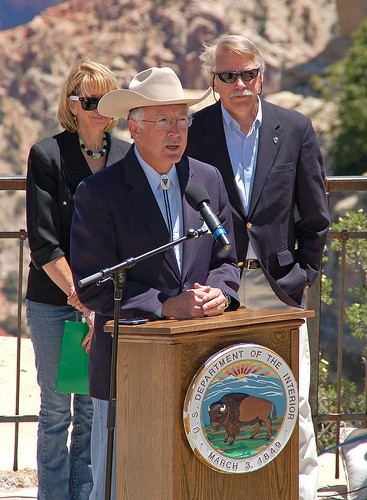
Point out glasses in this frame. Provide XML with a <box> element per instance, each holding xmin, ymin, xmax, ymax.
<box><xmin>67</xmin><ymin>95</ymin><xmax>100</xmax><ymax>111</ymax></box>
<box><xmin>213</xmin><ymin>65</ymin><xmax>261</xmax><ymax>83</ymax></box>
<box><xmin>141</xmin><ymin>116</ymin><xmax>195</xmax><ymax>128</ymax></box>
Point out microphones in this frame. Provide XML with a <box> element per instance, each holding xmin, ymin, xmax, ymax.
<box><xmin>185</xmin><ymin>182</ymin><xmax>232</xmax><ymax>252</ymax></box>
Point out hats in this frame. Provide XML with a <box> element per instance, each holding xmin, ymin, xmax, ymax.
<box><xmin>97</xmin><ymin>67</ymin><xmax>213</xmax><ymax>118</ymax></box>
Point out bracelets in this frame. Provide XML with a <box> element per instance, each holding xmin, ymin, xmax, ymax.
<box><xmin>81</xmin><ymin>311</ymin><xmax>94</xmax><ymax>324</ymax></box>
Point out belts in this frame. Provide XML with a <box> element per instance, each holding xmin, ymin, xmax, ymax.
<box><xmin>244</xmin><ymin>257</ymin><xmax>261</xmax><ymax>270</ymax></box>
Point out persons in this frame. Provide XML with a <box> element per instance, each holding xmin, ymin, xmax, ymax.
<box><xmin>26</xmin><ymin>62</ymin><xmax>132</xmax><ymax>500</ymax></box>
<box><xmin>71</xmin><ymin>67</ymin><xmax>241</xmax><ymax>500</ymax></box>
<box><xmin>186</xmin><ymin>34</ymin><xmax>330</xmax><ymax>500</ymax></box>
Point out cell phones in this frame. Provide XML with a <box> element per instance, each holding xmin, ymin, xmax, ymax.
<box><xmin>118</xmin><ymin>318</ymin><xmax>148</xmax><ymax>324</ymax></box>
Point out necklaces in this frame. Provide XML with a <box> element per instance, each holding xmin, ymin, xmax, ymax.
<box><xmin>78</xmin><ymin>133</ymin><xmax>108</xmax><ymax>160</ymax></box>
